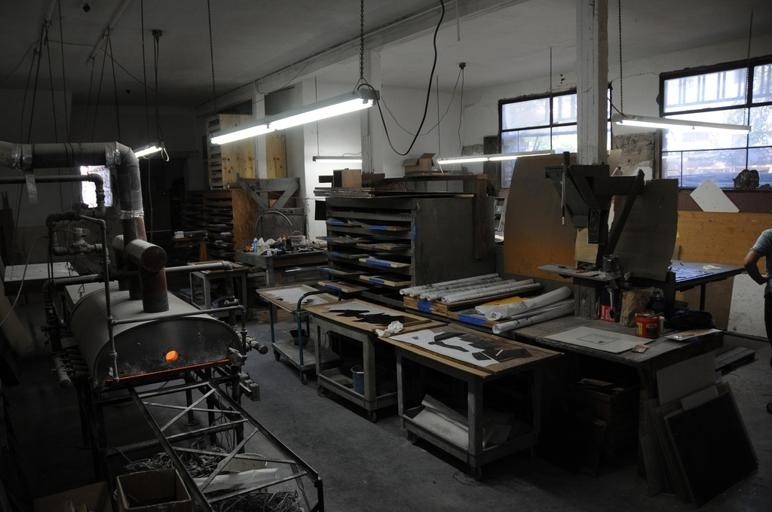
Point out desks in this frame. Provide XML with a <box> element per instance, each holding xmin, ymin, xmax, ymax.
<box><xmin>378</xmin><ymin>321</ymin><xmax>563</xmax><ymax>479</ymax></box>
<box><xmin>187</xmin><ymin>260</ymin><xmax>249</xmax><ymax>326</ymax></box>
<box><xmin>243</xmin><ymin>250</ymin><xmax>328</xmax><ymax>348</ymax></box>
<box><xmin>256</xmin><ymin>281</ymin><xmax>348</xmax><ymax>383</ymax></box>
<box><xmin>301</xmin><ymin>299</ymin><xmax>446</xmax><ymax>421</ymax></box>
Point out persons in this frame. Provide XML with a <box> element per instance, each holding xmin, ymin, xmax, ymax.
<box><xmin>743</xmin><ymin>228</ymin><xmax>772</xmax><ymax>413</ymax></box>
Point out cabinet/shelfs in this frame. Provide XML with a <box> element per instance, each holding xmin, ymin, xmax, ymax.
<box><xmin>319</xmin><ymin>188</ymin><xmax>496</xmax><ymax>310</ymax></box>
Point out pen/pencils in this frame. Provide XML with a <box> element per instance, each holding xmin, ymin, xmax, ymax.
<box><xmin>495</xmin><ymin>349</ymin><xmax>503</xmax><ymax>356</ymax></box>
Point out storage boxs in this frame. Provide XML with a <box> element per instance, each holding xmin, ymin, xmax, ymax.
<box><xmin>115</xmin><ymin>468</ymin><xmax>193</xmax><ymax>512</ymax></box>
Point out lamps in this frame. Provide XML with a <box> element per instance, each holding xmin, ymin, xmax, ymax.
<box><xmin>436</xmin><ymin>150</ymin><xmax>558</xmax><ymax>164</ymax></box>
<box><xmin>613</xmin><ymin>113</ymin><xmax>751</xmax><ymax>135</ymax></box>
<box><xmin>135</xmin><ymin>140</ymin><xmax>166</xmax><ymax>158</ymax></box>
<box><xmin>312</xmin><ymin>155</ymin><xmax>366</xmax><ymax>161</ymax></box>
<box><xmin>208</xmin><ymin>89</ymin><xmax>380</xmax><ymax>145</ymax></box>
